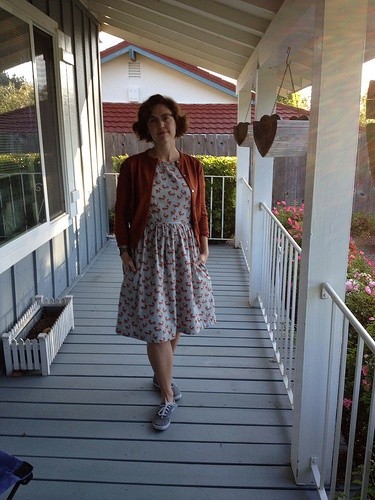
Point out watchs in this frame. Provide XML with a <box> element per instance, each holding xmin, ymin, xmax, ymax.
<box><xmin>117</xmin><ymin>247</ymin><xmax>128</xmax><ymax>255</ymax></box>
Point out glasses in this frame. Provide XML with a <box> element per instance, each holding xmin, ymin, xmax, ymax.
<box><xmin>145</xmin><ymin>112</ymin><xmax>176</xmax><ymax>124</ymax></box>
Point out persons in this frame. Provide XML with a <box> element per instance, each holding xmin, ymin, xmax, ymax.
<box><xmin>115</xmin><ymin>94</ymin><xmax>217</xmax><ymax>429</ymax></box>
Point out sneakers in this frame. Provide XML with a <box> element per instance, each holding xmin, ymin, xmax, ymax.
<box><xmin>152</xmin><ymin>372</ymin><xmax>183</xmax><ymax>400</ymax></box>
<box><xmin>152</xmin><ymin>399</ymin><xmax>179</xmax><ymax>431</ymax></box>
